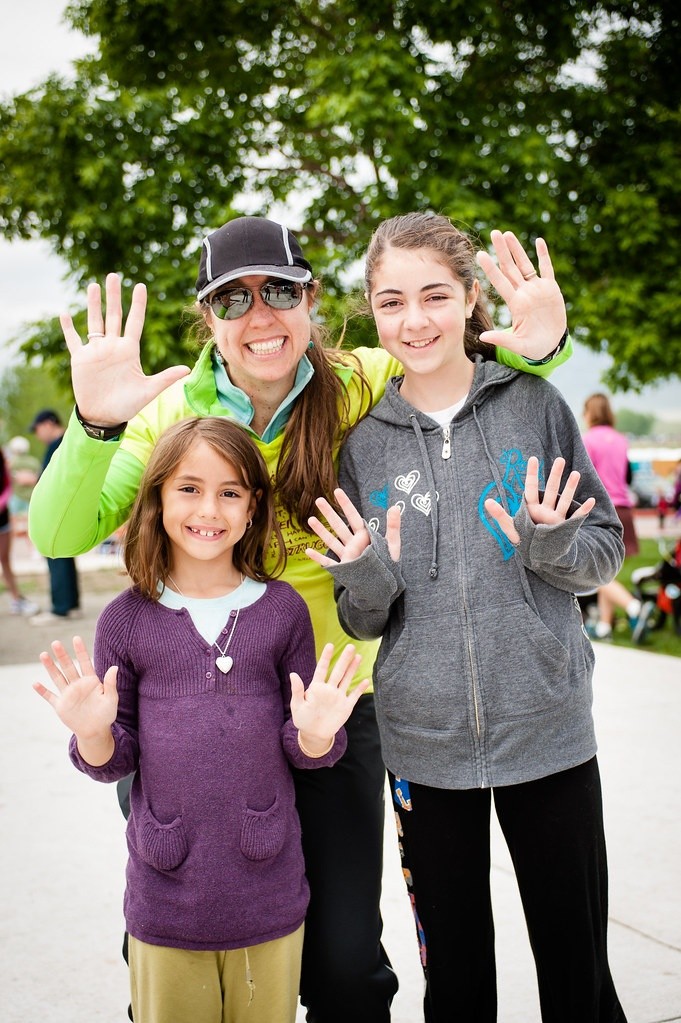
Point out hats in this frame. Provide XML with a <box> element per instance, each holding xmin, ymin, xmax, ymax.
<box><xmin>31</xmin><ymin>411</ymin><xmax>60</xmax><ymax>433</ymax></box>
<box><xmin>195</xmin><ymin>217</ymin><xmax>313</xmax><ymax>303</ymax></box>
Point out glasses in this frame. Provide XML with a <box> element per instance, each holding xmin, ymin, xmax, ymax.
<box><xmin>204</xmin><ymin>277</ymin><xmax>309</xmax><ymax>320</ymax></box>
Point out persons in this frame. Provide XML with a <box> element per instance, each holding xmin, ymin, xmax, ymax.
<box><xmin>28</xmin><ymin>215</ymin><xmax>573</xmax><ymax>1023</ymax></box>
<box><xmin>29</xmin><ymin>409</ymin><xmax>81</xmax><ymax>627</ymax></box>
<box><xmin>581</xmin><ymin>395</ymin><xmax>658</xmax><ymax>644</ymax></box>
<box><xmin>304</xmin><ymin>212</ymin><xmax>629</xmax><ymax>1023</ymax></box>
<box><xmin>0</xmin><ymin>448</ymin><xmax>40</xmax><ymax>618</ymax></box>
<box><xmin>32</xmin><ymin>417</ymin><xmax>371</xmax><ymax>1023</ymax></box>
<box><xmin>6</xmin><ymin>436</ymin><xmax>43</xmax><ymax>537</ymax></box>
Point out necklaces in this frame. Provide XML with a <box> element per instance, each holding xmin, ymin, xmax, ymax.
<box><xmin>167</xmin><ymin>571</ymin><xmax>244</xmax><ymax>675</ymax></box>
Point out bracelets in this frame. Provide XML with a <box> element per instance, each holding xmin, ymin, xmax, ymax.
<box><xmin>75</xmin><ymin>406</ymin><xmax>127</xmax><ymax>440</ymax></box>
<box><xmin>298</xmin><ymin>730</ymin><xmax>335</xmax><ymax>758</ymax></box>
<box><xmin>521</xmin><ymin>331</ymin><xmax>568</xmax><ymax>366</ymax></box>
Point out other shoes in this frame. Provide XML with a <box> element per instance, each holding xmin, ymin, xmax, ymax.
<box><xmin>67</xmin><ymin>608</ymin><xmax>84</xmax><ymax>620</ymax></box>
<box><xmin>9</xmin><ymin>597</ymin><xmax>40</xmax><ymax>616</ymax></box>
<box><xmin>582</xmin><ymin>622</ymin><xmax>614</xmax><ymax>641</ymax></box>
<box><xmin>30</xmin><ymin>608</ymin><xmax>67</xmax><ymax>625</ymax></box>
<box><xmin>627</xmin><ymin>601</ymin><xmax>654</xmax><ymax>644</ymax></box>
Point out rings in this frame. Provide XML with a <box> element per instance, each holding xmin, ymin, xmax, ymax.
<box><xmin>523</xmin><ymin>270</ymin><xmax>536</xmax><ymax>279</ymax></box>
<box><xmin>88</xmin><ymin>333</ymin><xmax>104</xmax><ymax>340</ymax></box>
